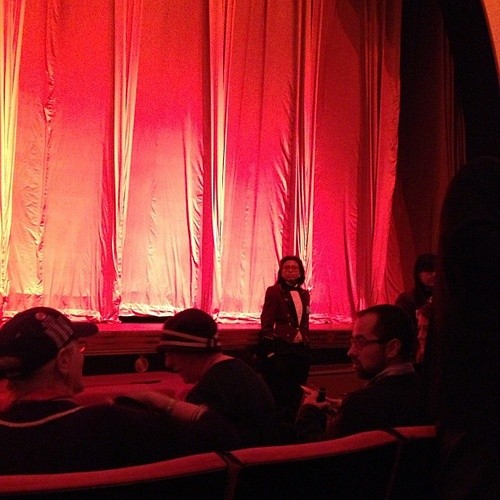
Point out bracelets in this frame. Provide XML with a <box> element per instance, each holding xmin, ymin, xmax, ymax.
<box><xmin>168</xmin><ymin>398</ymin><xmax>176</xmax><ymax>414</ymax></box>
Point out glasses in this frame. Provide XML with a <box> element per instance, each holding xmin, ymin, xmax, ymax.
<box><xmin>59</xmin><ymin>341</ymin><xmax>86</xmax><ymax>352</ymax></box>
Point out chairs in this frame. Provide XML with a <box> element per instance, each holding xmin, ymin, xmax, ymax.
<box><xmin>0</xmin><ymin>420</ymin><xmax>437</xmax><ymax>500</ymax></box>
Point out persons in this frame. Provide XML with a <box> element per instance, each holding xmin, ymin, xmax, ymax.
<box><xmin>394</xmin><ymin>254</ymin><xmax>438</xmax><ymax>366</ymax></box>
<box><xmin>415</xmin><ymin>296</ymin><xmax>433</xmax><ymax>364</ymax></box>
<box><xmin>298</xmin><ymin>304</ymin><xmax>449</xmax><ymax>442</ymax></box>
<box><xmin>260</xmin><ymin>256</ymin><xmax>310</xmax><ymax>422</ymax></box>
<box><xmin>0</xmin><ymin>307</ymin><xmax>166</xmax><ymax>477</ymax></box>
<box><xmin>136</xmin><ymin>309</ymin><xmax>277</xmax><ymax>456</ymax></box>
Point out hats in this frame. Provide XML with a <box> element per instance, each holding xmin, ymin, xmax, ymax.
<box><xmin>0</xmin><ymin>307</ymin><xmax>98</xmax><ymax>374</ymax></box>
<box><xmin>158</xmin><ymin>308</ymin><xmax>222</xmax><ymax>352</ymax></box>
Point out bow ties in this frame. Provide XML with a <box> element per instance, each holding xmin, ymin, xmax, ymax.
<box><xmin>288</xmin><ymin>285</ymin><xmax>300</xmax><ymax>291</ymax></box>
